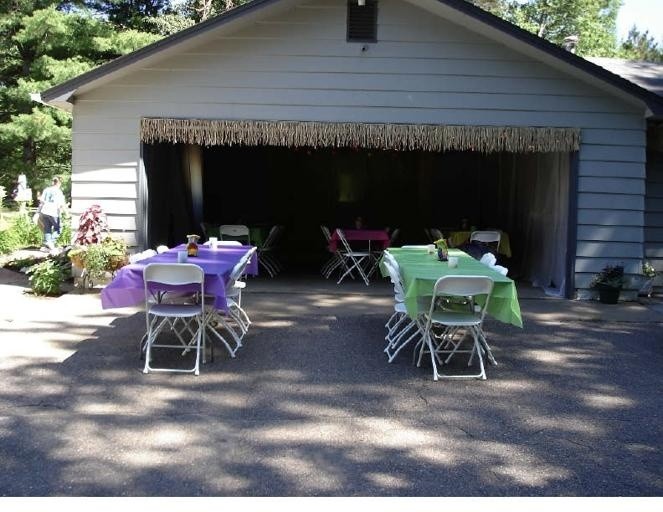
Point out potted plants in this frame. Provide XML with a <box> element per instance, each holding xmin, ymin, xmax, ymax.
<box><xmin>585</xmin><ymin>261</ymin><xmax>627</xmax><ymax>304</ymax></box>
<box><xmin>68</xmin><ymin>237</ymin><xmax>131</xmax><ymax>293</ymax></box>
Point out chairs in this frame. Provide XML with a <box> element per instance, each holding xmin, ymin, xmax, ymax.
<box><xmin>126</xmin><ymin>222</ymin><xmax>284</xmax><ymax>360</ymax></box>
<box><xmin>422</xmin><ymin>273</ymin><xmax>497</xmax><ymax>382</ymax></box>
<box><xmin>141</xmin><ymin>262</ymin><xmax>210</xmax><ymax>377</ymax></box>
<box><xmin>320</xmin><ymin>215</ymin><xmax>522</xmax><ymax>365</ymax></box>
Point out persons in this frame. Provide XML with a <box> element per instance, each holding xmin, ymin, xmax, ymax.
<box><xmin>36</xmin><ymin>177</ymin><xmax>69</xmax><ymax>245</ymax></box>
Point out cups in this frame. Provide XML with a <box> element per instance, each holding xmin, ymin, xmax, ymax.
<box><xmin>447</xmin><ymin>257</ymin><xmax>457</xmax><ymax>267</ymax></box>
<box><xmin>426</xmin><ymin>244</ymin><xmax>435</xmax><ymax>253</ymax></box>
<box><xmin>208</xmin><ymin>237</ymin><xmax>217</xmax><ymax>249</ymax></box>
<box><xmin>177</xmin><ymin>250</ymin><xmax>188</xmax><ymax>263</ymax></box>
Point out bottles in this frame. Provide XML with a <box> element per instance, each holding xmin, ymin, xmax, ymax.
<box><xmin>186</xmin><ymin>235</ymin><xmax>198</xmax><ymax>256</ymax></box>
